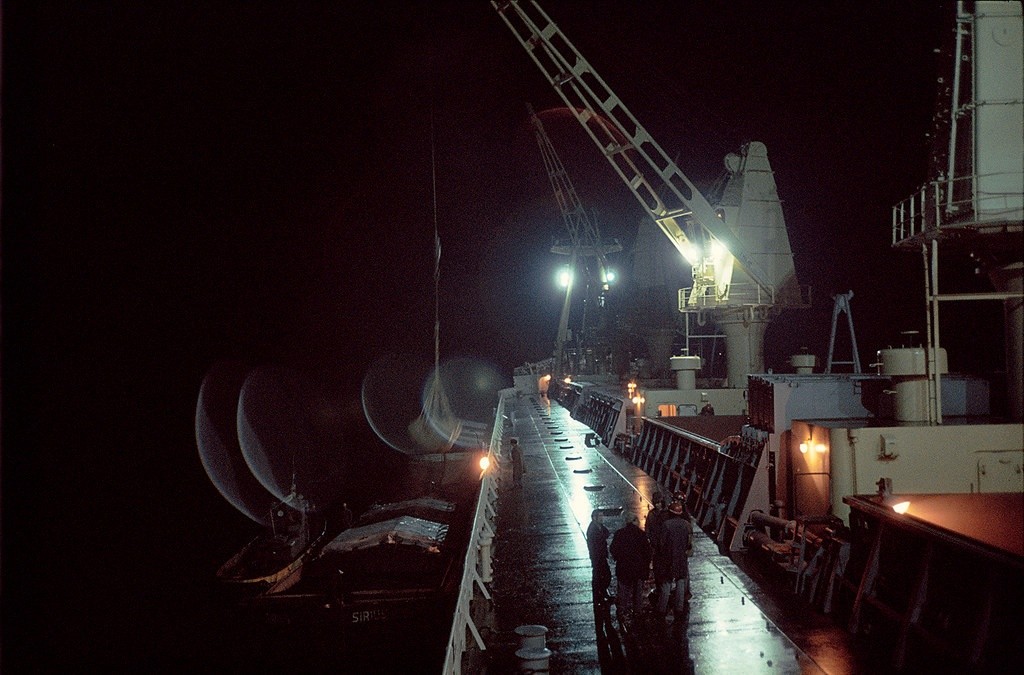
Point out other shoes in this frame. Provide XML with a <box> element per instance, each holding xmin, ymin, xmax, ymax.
<box><xmin>684</xmin><ymin>593</ymin><xmax>692</xmax><ymax>601</ymax></box>
<box><xmin>511</xmin><ymin>485</ymin><xmax>522</xmax><ymax>490</ymax></box>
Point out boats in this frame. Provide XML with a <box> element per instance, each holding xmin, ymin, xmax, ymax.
<box><xmin>216</xmin><ymin>454</ymin><xmax>468</xmax><ymax>651</ymax></box>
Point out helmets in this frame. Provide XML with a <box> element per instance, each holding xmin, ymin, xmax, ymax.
<box><xmin>651</xmin><ymin>492</ymin><xmax>665</xmax><ymax>503</ymax></box>
<box><xmin>625</xmin><ymin>512</ymin><xmax>637</xmax><ymax>523</ymax></box>
<box><xmin>666</xmin><ymin>501</ymin><xmax>682</xmax><ymax>514</ymax></box>
<box><xmin>669</xmin><ymin>490</ymin><xmax>687</xmax><ymax>505</ymax></box>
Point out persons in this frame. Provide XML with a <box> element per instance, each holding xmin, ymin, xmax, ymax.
<box><xmin>610</xmin><ymin>507</ymin><xmax>653</xmax><ymax>624</ymax></box>
<box><xmin>700</xmin><ymin>404</ymin><xmax>714</xmax><ymax>415</ymax></box>
<box><xmin>645</xmin><ymin>492</ymin><xmax>694</xmax><ymax>619</ymax></box>
<box><xmin>510</xmin><ymin>439</ymin><xmax>524</xmax><ymax>491</ymax></box>
<box><xmin>586</xmin><ymin>509</ymin><xmax>615</xmax><ymax>606</ymax></box>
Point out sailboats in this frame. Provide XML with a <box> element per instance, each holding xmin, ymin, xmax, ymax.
<box><xmin>404</xmin><ymin>108</ymin><xmax>494</xmax><ymax>453</ymax></box>
